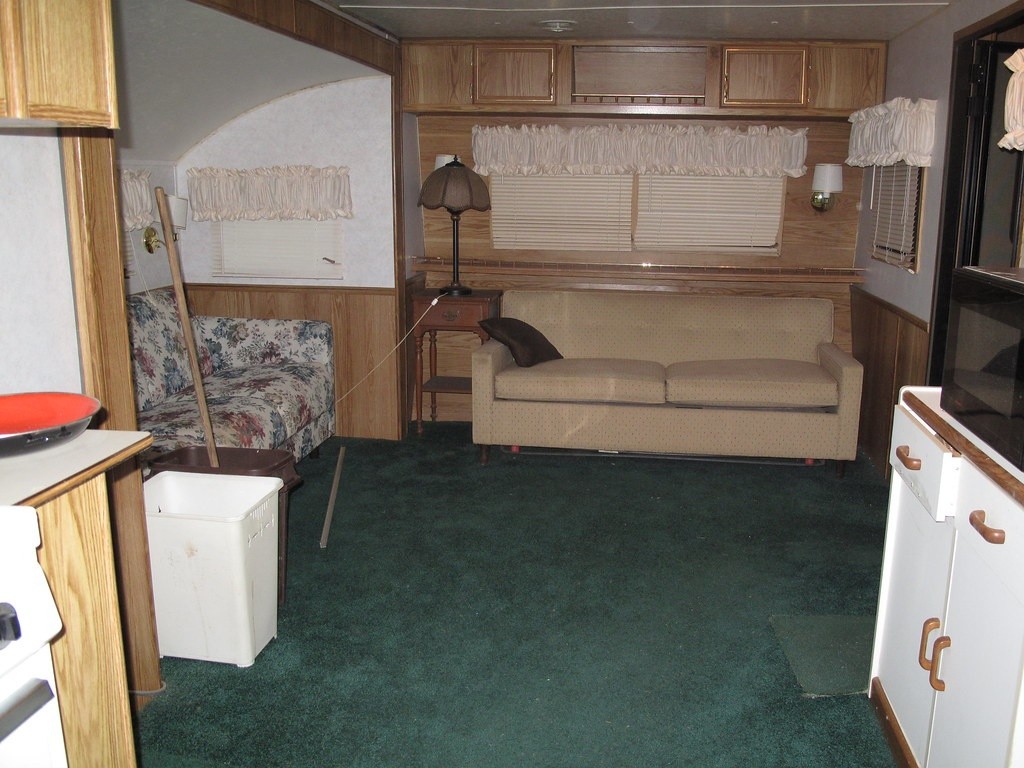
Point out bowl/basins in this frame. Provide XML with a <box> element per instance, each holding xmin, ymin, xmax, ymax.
<box><xmin>0</xmin><ymin>392</ymin><xmax>100</xmax><ymax>458</ymax></box>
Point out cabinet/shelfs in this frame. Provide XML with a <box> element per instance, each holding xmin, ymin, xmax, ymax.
<box><xmin>719</xmin><ymin>38</ymin><xmax>888</xmax><ymax>116</ymax></box>
<box><xmin>867</xmin><ymin>0</ymin><xmax>1024</xmax><ymax>768</ymax></box>
<box><xmin>400</xmin><ymin>38</ymin><xmax>556</xmax><ymax>112</ymax></box>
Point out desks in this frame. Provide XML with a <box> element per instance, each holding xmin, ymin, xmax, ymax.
<box><xmin>413</xmin><ymin>290</ymin><xmax>501</xmax><ymax>435</ymax></box>
<box><xmin>0</xmin><ymin>430</ymin><xmax>154</xmax><ymax>768</ymax></box>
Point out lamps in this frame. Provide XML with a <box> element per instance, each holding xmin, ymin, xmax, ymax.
<box><xmin>143</xmin><ymin>193</ymin><xmax>189</xmax><ymax>253</ymax></box>
<box><xmin>812</xmin><ymin>162</ymin><xmax>843</xmax><ymax>211</ymax></box>
<box><xmin>417</xmin><ymin>154</ymin><xmax>492</xmax><ymax>295</ymax></box>
<box><xmin>435</xmin><ymin>154</ymin><xmax>462</xmax><ymax>171</ymax></box>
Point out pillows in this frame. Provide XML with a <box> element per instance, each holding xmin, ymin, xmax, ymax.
<box><xmin>479</xmin><ymin>317</ymin><xmax>563</xmax><ymax>367</ymax></box>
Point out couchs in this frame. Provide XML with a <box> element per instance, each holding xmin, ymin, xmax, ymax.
<box><xmin>470</xmin><ymin>290</ymin><xmax>865</xmax><ymax>466</ymax></box>
<box><xmin>125</xmin><ymin>287</ymin><xmax>335</xmax><ymax>465</ymax></box>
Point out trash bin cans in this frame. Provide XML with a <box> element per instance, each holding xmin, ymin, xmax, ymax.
<box><xmin>142</xmin><ymin>471</ymin><xmax>284</xmax><ymax>668</ymax></box>
<box><xmin>149</xmin><ymin>445</ymin><xmax>302</xmax><ymax>608</ymax></box>
